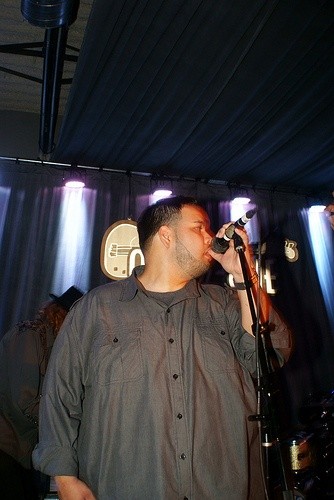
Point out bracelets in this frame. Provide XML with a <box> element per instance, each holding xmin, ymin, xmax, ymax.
<box><xmin>233</xmin><ymin>271</ymin><xmax>258</xmax><ymax>290</ymax></box>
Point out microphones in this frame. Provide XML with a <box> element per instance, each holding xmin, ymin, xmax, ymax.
<box><xmin>211</xmin><ymin>209</ymin><xmax>255</xmax><ymax>253</ymax></box>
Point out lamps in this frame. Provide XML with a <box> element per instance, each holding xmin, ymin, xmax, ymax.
<box><xmin>20</xmin><ymin>0</ymin><xmax>81</xmax><ymax>29</ymax></box>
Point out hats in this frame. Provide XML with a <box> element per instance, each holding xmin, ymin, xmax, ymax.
<box><xmin>49</xmin><ymin>284</ymin><xmax>83</xmax><ymax>311</ymax></box>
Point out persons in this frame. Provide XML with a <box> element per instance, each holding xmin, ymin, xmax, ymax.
<box><xmin>32</xmin><ymin>194</ymin><xmax>293</xmax><ymax>500</ymax></box>
<box><xmin>325</xmin><ymin>204</ymin><xmax>334</xmax><ymax>238</ymax></box>
<box><xmin>0</xmin><ymin>287</ymin><xmax>84</xmax><ymax>500</ymax></box>
